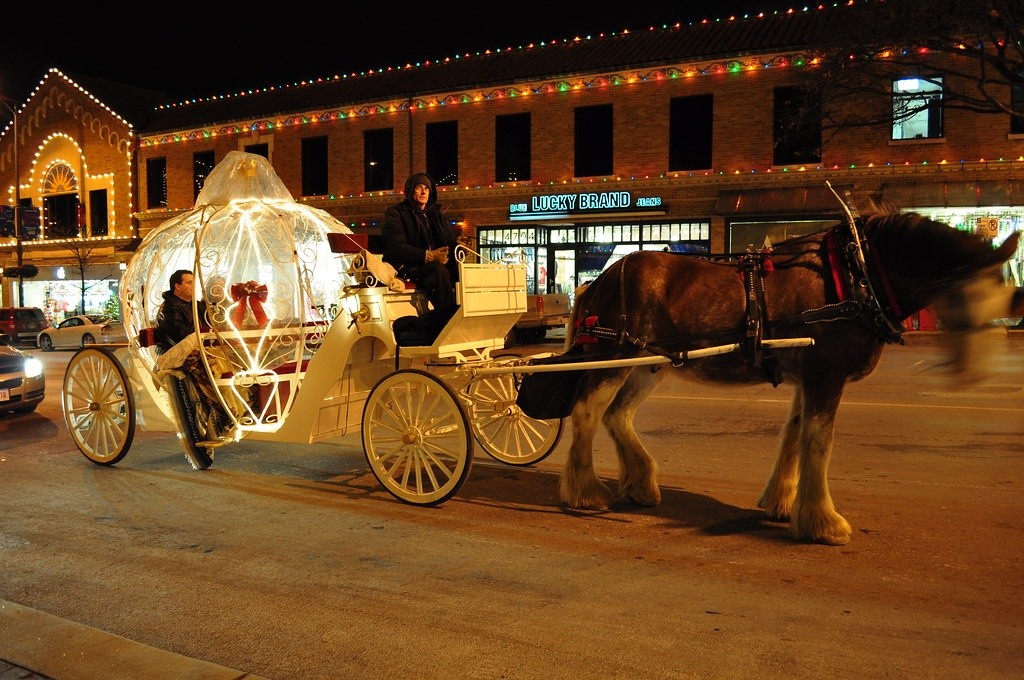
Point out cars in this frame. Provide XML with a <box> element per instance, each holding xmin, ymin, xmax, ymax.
<box><xmin>35</xmin><ymin>313</ymin><xmax>130</xmax><ymax>352</ymax></box>
<box><xmin>0</xmin><ymin>340</ymin><xmax>46</xmax><ymax>416</ymax></box>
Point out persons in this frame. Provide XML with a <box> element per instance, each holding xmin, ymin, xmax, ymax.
<box><xmin>380</xmin><ymin>171</ymin><xmax>475</xmax><ymax>309</ymax></box>
<box><xmin>153</xmin><ymin>269</ymin><xmax>264</xmax><ymax>432</ymax></box>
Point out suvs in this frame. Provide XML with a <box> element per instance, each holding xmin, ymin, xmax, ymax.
<box><xmin>0</xmin><ymin>306</ymin><xmax>49</xmax><ymax>346</ymax></box>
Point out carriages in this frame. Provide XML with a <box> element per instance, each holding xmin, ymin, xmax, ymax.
<box><xmin>59</xmin><ymin>192</ymin><xmax>1021</xmax><ymax>547</ymax></box>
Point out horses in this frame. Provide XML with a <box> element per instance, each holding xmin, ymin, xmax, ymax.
<box><xmin>558</xmin><ymin>209</ymin><xmax>1024</xmax><ymax>547</ymax></box>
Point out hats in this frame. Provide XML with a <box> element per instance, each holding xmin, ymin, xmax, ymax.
<box><xmin>415</xmin><ymin>175</ymin><xmax>431</xmax><ymax>189</ymax></box>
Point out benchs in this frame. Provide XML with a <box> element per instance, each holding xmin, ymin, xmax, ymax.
<box><xmin>328</xmin><ymin>233</ymin><xmax>456</xmax><ymax>292</ymax></box>
<box><xmin>140</xmin><ymin>319</ymin><xmax>328</xmax><ymax>416</ymax></box>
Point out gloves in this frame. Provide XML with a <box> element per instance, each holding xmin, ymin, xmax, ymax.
<box><xmin>428</xmin><ymin>247</ymin><xmax>449</xmax><ymax>265</ymax></box>
<box><xmin>458</xmin><ymin>235</ymin><xmax>473</xmax><ymax>255</ymax></box>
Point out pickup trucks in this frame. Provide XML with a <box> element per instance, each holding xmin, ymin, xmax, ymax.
<box><xmin>501</xmin><ymin>291</ymin><xmax>571</xmax><ymax>350</ymax></box>
